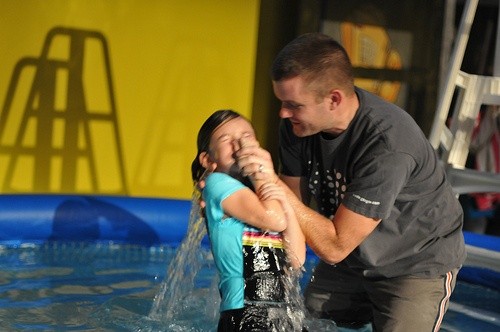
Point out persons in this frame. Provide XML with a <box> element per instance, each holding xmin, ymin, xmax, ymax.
<box><xmin>191</xmin><ymin>108</ymin><xmax>307</xmax><ymax>331</ymax></box>
<box><xmin>194</xmin><ymin>32</ymin><xmax>468</xmax><ymax>331</ymax></box>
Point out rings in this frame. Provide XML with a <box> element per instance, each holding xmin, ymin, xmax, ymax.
<box><xmin>258</xmin><ymin>163</ymin><xmax>266</xmax><ymax>172</ymax></box>
